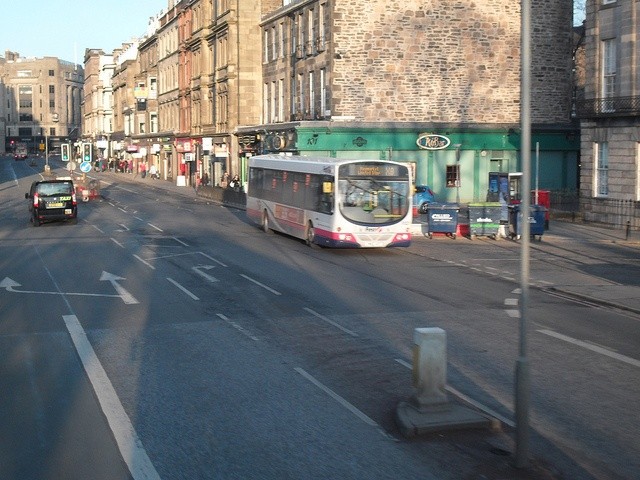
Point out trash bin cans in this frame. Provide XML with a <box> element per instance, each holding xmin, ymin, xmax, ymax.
<box><xmin>511</xmin><ymin>204</ymin><xmax>548</xmax><ymax>241</ymax></box>
<box><xmin>467</xmin><ymin>202</ymin><xmax>503</xmax><ymax>241</ymax></box>
<box><xmin>425</xmin><ymin>203</ymin><xmax>460</xmax><ymax>240</ymax></box>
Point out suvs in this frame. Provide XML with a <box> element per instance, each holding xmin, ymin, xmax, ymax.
<box><xmin>414</xmin><ymin>185</ymin><xmax>438</xmax><ymax>214</ymax></box>
<box><xmin>25</xmin><ymin>179</ymin><xmax>79</xmax><ymax>227</ymax></box>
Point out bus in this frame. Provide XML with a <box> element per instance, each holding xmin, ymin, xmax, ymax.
<box><xmin>245</xmin><ymin>154</ymin><xmax>413</xmax><ymax>250</ymax></box>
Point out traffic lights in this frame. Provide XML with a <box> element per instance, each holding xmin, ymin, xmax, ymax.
<box><xmin>60</xmin><ymin>143</ymin><xmax>70</xmax><ymax>162</ymax></box>
<box><xmin>82</xmin><ymin>142</ymin><xmax>92</xmax><ymax>163</ymax></box>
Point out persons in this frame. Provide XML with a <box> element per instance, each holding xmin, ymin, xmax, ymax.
<box><xmin>202</xmin><ymin>169</ymin><xmax>208</xmax><ymax>187</ymax></box>
<box><xmin>108</xmin><ymin>155</ymin><xmax>133</xmax><ymax>175</ymax></box>
<box><xmin>149</xmin><ymin>164</ymin><xmax>157</xmax><ymax>181</ymax></box>
<box><xmin>233</xmin><ymin>174</ymin><xmax>240</xmax><ymax>187</ymax></box>
<box><xmin>139</xmin><ymin>160</ymin><xmax>147</xmax><ymax>179</ymax></box>
<box><xmin>221</xmin><ymin>171</ymin><xmax>230</xmax><ymax>182</ymax></box>
